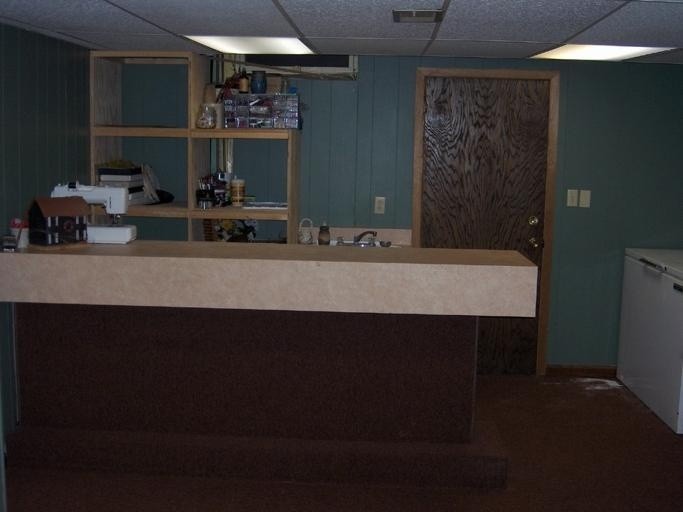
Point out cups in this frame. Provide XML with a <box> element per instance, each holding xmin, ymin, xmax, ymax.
<box><xmin>10</xmin><ymin>227</ymin><xmax>29</xmax><ymax>249</ymax></box>
<box><xmin>199</xmin><ymin>189</ymin><xmax>214</xmax><ymax>208</ymax></box>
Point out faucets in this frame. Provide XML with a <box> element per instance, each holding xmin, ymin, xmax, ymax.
<box><xmin>352</xmin><ymin>230</ymin><xmax>378</xmax><ymax>244</ymax></box>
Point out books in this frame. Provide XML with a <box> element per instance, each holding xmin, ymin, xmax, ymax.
<box><xmin>96</xmin><ymin>169</ymin><xmax>149</xmax><ymax>206</ymax></box>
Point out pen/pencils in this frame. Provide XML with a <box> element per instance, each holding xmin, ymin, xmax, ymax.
<box><xmin>198</xmin><ymin>180</ymin><xmax>210</xmax><ymax>210</ymax></box>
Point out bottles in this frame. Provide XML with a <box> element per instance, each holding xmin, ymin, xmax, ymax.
<box><xmin>317</xmin><ymin>223</ymin><xmax>330</xmax><ymax>245</ymax></box>
<box><xmin>223</xmin><ymin>76</ymin><xmax>237</xmax><ymax>128</ymax></box>
<box><xmin>282</xmin><ymin>78</ymin><xmax>289</xmax><ymax>92</ymax></box>
<box><xmin>239</xmin><ymin>65</ymin><xmax>267</xmax><ymax>95</ymax></box>
<box><xmin>196</xmin><ymin>81</ymin><xmax>226</xmax><ymax>129</ymax></box>
<box><xmin>214</xmin><ymin>167</ymin><xmax>246</xmax><ymax>208</ymax></box>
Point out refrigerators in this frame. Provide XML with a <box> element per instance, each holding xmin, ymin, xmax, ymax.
<box><xmin>613</xmin><ymin>247</ymin><xmax>683</xmax><ymax>435</ymax></box>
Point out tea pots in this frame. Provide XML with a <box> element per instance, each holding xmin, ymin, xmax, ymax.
<box><xmin>296</xmin><ymin>217</ymin><xmax>314</xmax><ymax>244</ymax></box>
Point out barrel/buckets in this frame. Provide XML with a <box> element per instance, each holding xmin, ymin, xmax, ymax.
<box><xmin>296</xmin><ymin>217</ymin><xmax>320</xmax><ymax>244</ymax></box>
<box><xmin>9</xmin><ymin>217</ymin><xmax>30</xmax><ymax>250</ymax></box>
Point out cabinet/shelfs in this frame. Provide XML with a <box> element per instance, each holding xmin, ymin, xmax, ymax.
<box><xmin>86</xmin><ymin>48</ymin><xmax>300</xmax><ymax>243</ymax></box>
